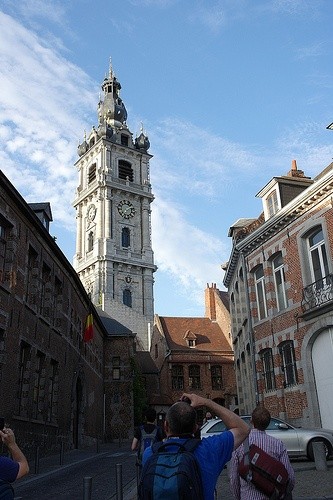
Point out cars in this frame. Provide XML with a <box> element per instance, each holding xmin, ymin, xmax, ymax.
<box><xmin>200</xmin><ymin>415</ymin><xmax>333</xmax><ymax>462</ymax></box>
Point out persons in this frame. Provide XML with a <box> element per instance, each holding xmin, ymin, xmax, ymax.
<box><xmin>230</xmin><ymin>406</ymin><xmax>295</xmax><ymax>500</ymax></box>
<box><xmin>131</xmin><ymin>407</ymin><xmax>162</xmax><ymax>474</ymax></box>
<box><xmin>203</xmin><ymin>410</ymin><xmax>216</xmax><ymax>424</ymax></box>
<box><xmin>0</xmin><ymin>416</ymin><xmax>29</xmax><ymax>500</ymax></box>
<box><xmin>137</xmin><ymin>393</ymin><xmax>250</xmax><ymax>500</ymax></box>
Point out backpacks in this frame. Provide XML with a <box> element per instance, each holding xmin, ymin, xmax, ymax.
<box><xmin>136</xmin><ymin>439</ymin><xmax>203</xmax><ymax>500</ymax></box>
<box><xmin>135</xmin><ymin>425</ymin><xmax>159</xmax><ymax>469</ymax></box>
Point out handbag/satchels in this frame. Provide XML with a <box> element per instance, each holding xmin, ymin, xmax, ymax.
<box><xmin>237</xmin><ymin>444</ymin><xmax>289</xmax><ymax>500</ymax></box>
